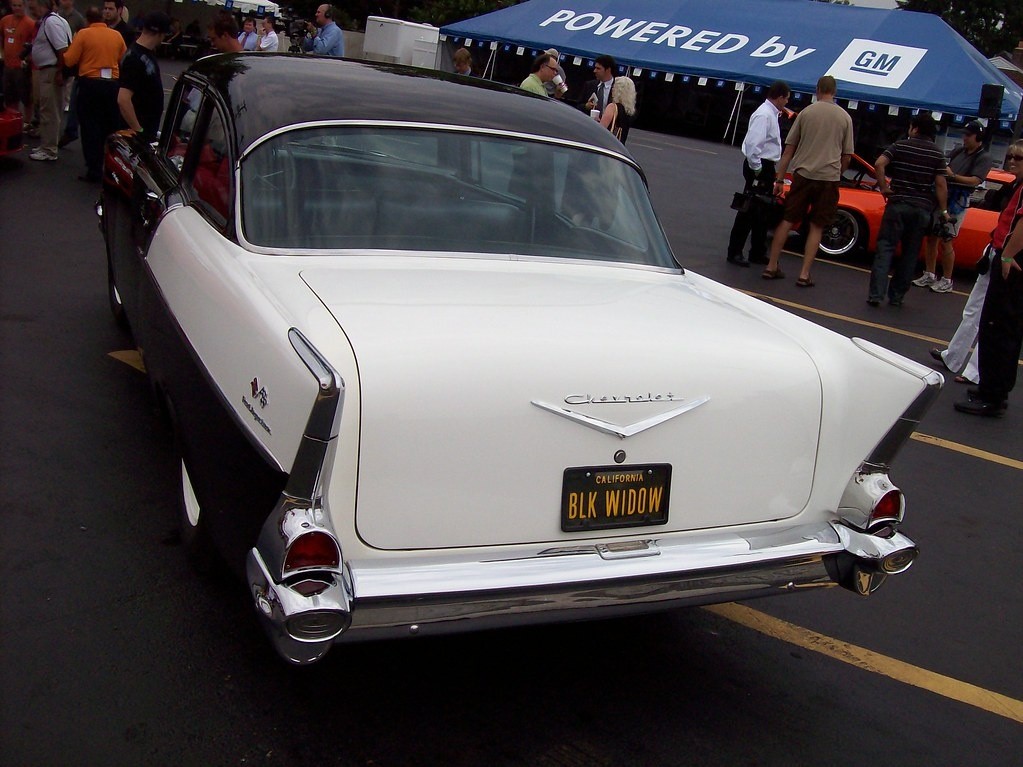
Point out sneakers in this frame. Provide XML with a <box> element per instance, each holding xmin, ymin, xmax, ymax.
<box><xmin>929</xmin><ymin>277</ymin><xmax>954</xmax><ymax>293</ymax></box>
<box><xmin>30</xmin><ymin>146</ymin><xmax>57</xmax><ymax>160</ymax></box>
<box><xmin>911</xmin><ymin>271</ymin><xmax>937</xmax><ymax>287</ymax></box>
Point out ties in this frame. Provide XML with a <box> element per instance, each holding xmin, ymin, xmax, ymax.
<box><xmin>595</xmin><ymin>82</ymin><xmax>605</xmax><ymax>116</ymax></box>
<box><xmin>241</xmin><ymin>34</ymin><xmax>248</xmax><ymax>47</ymax></box>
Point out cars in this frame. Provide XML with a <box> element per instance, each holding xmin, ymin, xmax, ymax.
<box><xmin>96</xmin><ymin>51</ymin><xmax>946</xmax><ymax>666</ymax></box>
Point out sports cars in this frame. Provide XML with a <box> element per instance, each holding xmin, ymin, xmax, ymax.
<box><xmin>761</xmin><ymin>153</ymin><xmax>1017</xmax><ymax>266</ymax></box>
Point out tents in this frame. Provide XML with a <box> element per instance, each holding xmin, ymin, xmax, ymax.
<box><xmin>171</xmin><ymin>0</ymin><xmax>284</xmax><ymax>45</ymax></box>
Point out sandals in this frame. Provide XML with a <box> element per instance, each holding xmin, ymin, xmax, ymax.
<box><xmin>762</xmin><ymin>267</ymin><xmax>784</xmax><ymax>279</ymax></box>
<box><xmin>953</xmin><ymin>375</ymin><xmax>973</xmax><ymax>383</ymax></box>
<box><xmin>796</xmin><ymin>271</ymin><xmax>816</xmax><ymax>287</ymax></box>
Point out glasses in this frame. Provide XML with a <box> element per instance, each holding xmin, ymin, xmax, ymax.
<box><xmin>546</xmin><ymin>64</ymin><xmax>559</xmax><ymax>72</ymax></box>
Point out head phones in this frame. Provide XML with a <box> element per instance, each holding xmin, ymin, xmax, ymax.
<box><xmin>324</xmin><ymin>3</ymin><xmax>332</xmax><ymax>18</ymax></box>
<box><xmin>973</xmin><ymin>120</ymin><xmax>985</xmax><ymax>142</ymax></box>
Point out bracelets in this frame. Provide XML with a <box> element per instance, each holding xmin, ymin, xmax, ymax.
<box><xmin>1001</xmin><ymin>256</ymin><xmax>1013</xmax><ymax>263</ymax></box>
<box><xmin>258</xmin><ymin>35</ymin><xmax>261</xmax><ymax>39</ymax></box>
<box><xmin>950</xmin><ymin>172</ymin><xmax>957</xmax><ymax>180</ymax></box>
<box><xmin>775</xmin><ymin>177</ymin><xmax>784</xmax><ymax>184</ymax></box>
<box><xmin>938</xmin><ymin>209</ymin><xmax>947</xmax><ymax>216</ymax></box>
<box><xmin>136</xmin><ymin>128</ymin><xmax>144</xmax><ymax>132</ymax></box>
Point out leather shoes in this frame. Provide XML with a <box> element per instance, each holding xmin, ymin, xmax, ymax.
<box><xmin>727</xmin><ymin>254</ymin><xmax>751</xmax><ymax>267</ymax></box>
<box><xmin>749</xmin><ymin>255</ymin><xmax>778</xmax><ymax>265</ymax></box>
<box><xmin>929</xmin><ymin>346</ymin><xmax>952</xmax><ymax>372</ymax></box>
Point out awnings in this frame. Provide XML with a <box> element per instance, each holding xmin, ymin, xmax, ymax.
<box><xmin>439</xmin><ymin>0</ymin><xmax>1023</xmax><ymax>148</ymax></box>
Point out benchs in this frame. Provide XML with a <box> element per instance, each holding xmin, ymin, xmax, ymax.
<box><xmin>243</xmin><ymin>189</ymin><xmax>528</xmax><ymax>251</ymax></box>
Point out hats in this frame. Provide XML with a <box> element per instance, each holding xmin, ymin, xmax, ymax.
<box><xmin>960</xmin><ymin>120</ymin><xmax>985</xmax><ymax>134</ymax></box>
<box><xmin>544</xmin><ymin>48</ymin><xmax>559</xmax><ymax>58</ymax></box>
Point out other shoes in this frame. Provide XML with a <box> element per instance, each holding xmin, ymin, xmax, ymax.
<box><xmin>77</xmin><ymin>171</ymin><xmax>102</xmax><ymax>184</ymax></box>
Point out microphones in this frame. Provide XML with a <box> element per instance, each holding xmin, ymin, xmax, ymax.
<box><xmin>941</xmin><ymin>216</ymin><xmax>958</xmax><ymax>224</ymax></box>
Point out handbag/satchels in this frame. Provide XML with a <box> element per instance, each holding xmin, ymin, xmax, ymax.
<box><xmin>730</xmin><ymin>185</ymin><xmax>769</xmax><ymax>215</ymax></box>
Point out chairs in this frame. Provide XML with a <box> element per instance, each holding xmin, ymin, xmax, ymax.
<box><xmin>977</xmin><ymin>188</ymin><xmax>1000</xmax><ymax>212</ymax></box>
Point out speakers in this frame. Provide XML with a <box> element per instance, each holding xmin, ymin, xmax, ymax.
<box><xmin>978</xmin><ymin>84</ymin><xmax>1005</xmax><ymax>118</ymax></box>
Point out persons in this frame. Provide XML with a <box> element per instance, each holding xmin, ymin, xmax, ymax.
<box><xmin>0</xmin><ymin>0</ymin><xmax>172</xmax><ymax>184</ymax></box>
<box><xmin>866</xmin><ymin>115</ymin><xmax>994</xmax><ymax>309</ymax></box>
<box><xmin>165</xmin><ymin>3</ymin><xmax>345</xmax><ymax>145</ymax></box>
<box><xmin>762</xmin><ymin>76</ymin><xmax>854</xmax><ymax>288</ymax></box>
<box><xmin>928</xmin><ymin>139</ymin><xmax>1023</xmax><ymax>418</ymax></box>
<box><xmin>437</xmin><ymin>48</ymin><xmax>483</xmax><ymax>173</ymax></box>
<box><xmin>727</xmin><ymin>80</ymin><xmax>790</xmax><ymax>267</ymax></box>
<box><xmin>507</xmin><ymin>48</ymin><xmax>637</xmax><ymax>229</ymax></box>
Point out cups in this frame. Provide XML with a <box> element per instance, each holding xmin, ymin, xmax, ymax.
<box><xmin>552</xmin><ymin>74</ymin><xmax>568</xmax><ymax>93</ymax></box>
<box><xmin>590</xmin><ymin>110</ymin><xmax>600</xmax><ymax>121</ymax></box>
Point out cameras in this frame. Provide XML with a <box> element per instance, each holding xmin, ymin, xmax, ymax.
<box><xmin>20</xmin><ymin>43</ymin><xmax>33</xmax><ymax>58</ymax></box>
<box><xmin>933</xmin><ymin>224</ymin><xmax>949</xmax><ymax>237</ymax></box>
